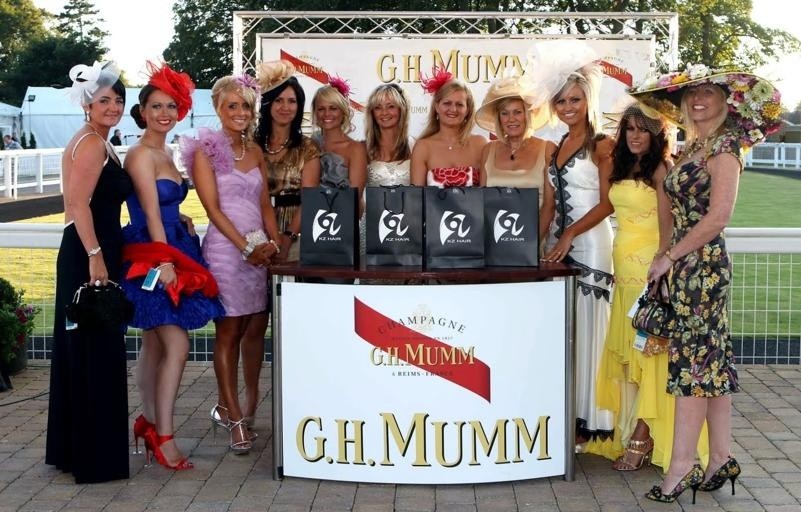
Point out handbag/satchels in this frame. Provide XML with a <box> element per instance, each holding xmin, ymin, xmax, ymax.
<box><xmin>87</xmin><ymin>245</ymin><xmax>101</xmax><ymax>258</ymax></box>
<box><xmin>241</xmin><ymin>241</ymin><xmax>254</xmax><ymax>258</ymax></box>
<box><xmin>269</xmin><ymin>238</ymin><xmax>281</xmax><ymax>254</ymax></box>
<box><xmin>666</xmin><ymin>249</ymin><xmax>677</xmax><ymax>264</ymax></box>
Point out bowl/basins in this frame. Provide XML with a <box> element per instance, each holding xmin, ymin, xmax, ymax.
<box><xmin>506</xmin><ymin>136</ymin><xmax>531</xmax><ymax>161</ymax></box>
<box><xmin>264</xmin><ymin>135</ymin><xmax>290</xmax><ymax>154</ymax></box>
<box><xmin>230</xmin><ymin>135</ymin><xmax>246</xmax><ymax>161</ymax></box>
<box><xmin>686</xmin><ymin>126</ymin><xmax>724</xmax><ymax>159</ymax></box>
<box><xmin>439</xmin><ymin>132</ymin><xmax>465</xmax><ymax>151</ymax></box>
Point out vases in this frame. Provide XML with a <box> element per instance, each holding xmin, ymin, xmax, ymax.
<box><xmin>0</xmin><ymin>277</ymin><xmax>42</xmax><ymax>363</ymax></box>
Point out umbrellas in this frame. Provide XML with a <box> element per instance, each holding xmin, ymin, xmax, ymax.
<box><xmin>576</xmin><ymin>435</ymin><xmax>593</xmax><ymax>453</ymax></box>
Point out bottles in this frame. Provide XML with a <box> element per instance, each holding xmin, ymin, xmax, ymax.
<box><xmin>474</xmin><ymin>78</ymin><xmax>551</xmax><ymax>132</ymax></box>
<box><xmin>626</xmin><ymin>63</ymin><xmax>782</xmax><ymax>150</ymax></box>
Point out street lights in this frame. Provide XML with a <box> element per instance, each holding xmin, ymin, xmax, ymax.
<box><xmin>67</xmin><ymin>279</ymin><xmax>131</xmax><ymax>337</ymax></box>
<box><xmin>298</xmin><ymin>184</ymin><xmax>360</xmax><ymax>269</ymax></box>
<box><xmin>632</xmin><ymin>276</ymin><xmax>678</xmax><ymax>342</ymax></box>
<box><xmin>364</xmin><ymin>184</ymin><xmax>541</xmax><ymax>271</ymax></box>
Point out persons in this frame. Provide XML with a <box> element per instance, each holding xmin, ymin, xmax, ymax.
<box><xmin>584</xmin><ymin>101</ymin><xmax>711</xmax><ymax>478</ymax></box>
<box><xmin>537</xmin><ymin>73</ymin><xmax>616</xmax><ymax>454</ymax></box>
<box><xmin>408</xmin><ymin>78</ymin><xmax>489</xmax><ymax>187</ymax></box>
<box><xmin>122</xmin><ymin>83</ymin><xmax>225</xmax><ymax>473</ymax></box>
<box><xmin>177</xmin><ymin>75</ymin><xmax>281</xmax><ymax>457</ymax></box>
<box><xmin>240</xmin><ymin>75</ymin><xmax>319</xmax><ymax>426</ymax></box>
<box><xmin>779</xmin><ymin>134</ymin><xmax>788</xmax><ymax>160</ymax></box>
<box><xmin>644</xmin><ymin>81</ymin><xmax>747</xmax><ymax>505</ymax></box>
<box><xmin>47</xmin><ymin>75</ymin><xmax>136</xmax><ymax>486</ymax></box>
<box><xmin>478</xmin><ymin>95</ymin><xmax>559</xmax><ymax>260</ymax></box>
<box><xmin>310</xmin><ymin>84</ymin><xmax>368</xmax><ymax>257</ymax></box>
<box><xmin>357</xmin><ymin>84</ymin><xmax>422</xmax><ymax>257</ymax></box>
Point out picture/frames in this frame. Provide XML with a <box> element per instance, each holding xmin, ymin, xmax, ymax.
<box><xmin>270</xmin><ymin>262</ymin><xmax>580</xmax><ymax>483</ymax></box>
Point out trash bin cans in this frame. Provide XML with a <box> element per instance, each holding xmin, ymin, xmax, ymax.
<box><xmin>285</xmin><ymin>228</ymin><xmax>298</xmax><ymax>243</ymax></box>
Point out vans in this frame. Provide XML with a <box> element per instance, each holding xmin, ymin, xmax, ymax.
<box><xmin>644</xmin><ymin>464</ymin><xmax>705</xmax><ymax>505</ymax></box>
<box><xmin>210</xmin><ymin>403</ymin><xmax>259</xmax><ymax>455</ymax></box>
<box><xmin>612</xmin><ymin>436</ymin><xmax>654</xmax><ymax>473</ymax></box>
<box><xmin>133</xmin><ymin>414</ymin><xmax>195</xmax><ymax>470</ymax></box>
<box><xmin>699</xmin><ymin>457</ymin><xmax>741</xmax><ymax>496</ymax></box>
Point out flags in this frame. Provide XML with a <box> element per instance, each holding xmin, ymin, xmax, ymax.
<box><xmin>5</xmin><ymin>338</ymin><xmax>29</xmax><ymax>376</ymax></box>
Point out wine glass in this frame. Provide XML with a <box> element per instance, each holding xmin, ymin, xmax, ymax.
<box><xmin>556</xmin><ymin>251</ymin><xmax>562</xmax><ymax>254</ymax></box>
